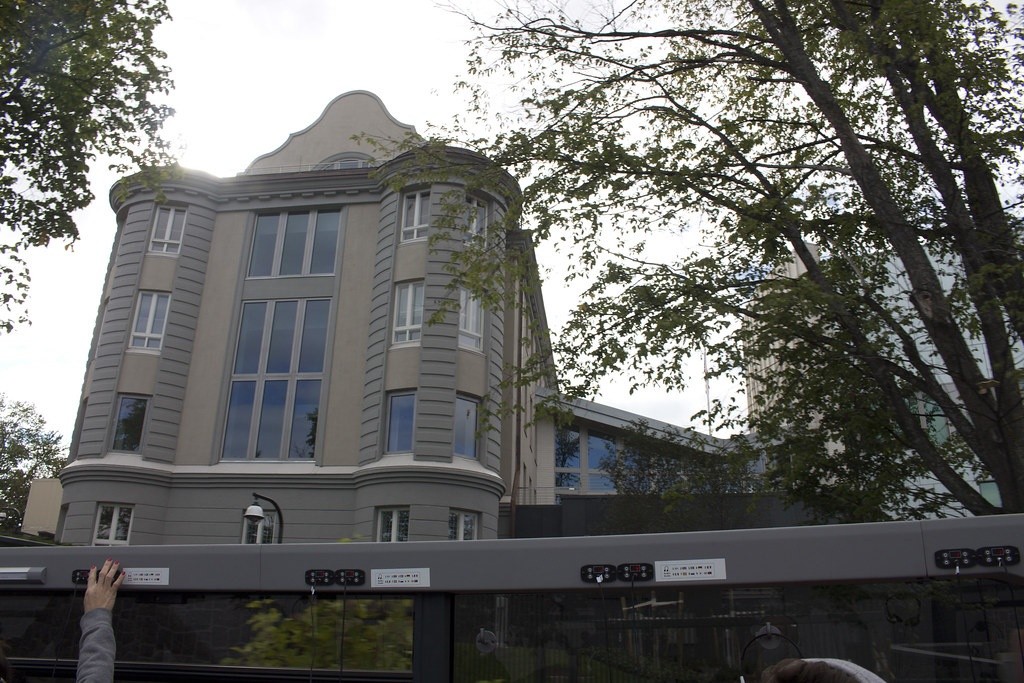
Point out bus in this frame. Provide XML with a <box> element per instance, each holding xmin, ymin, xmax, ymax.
<box><xmin>0</xmin><ymin>513</ymin><xmax>1024</xmax><ymax>683</ymax></box>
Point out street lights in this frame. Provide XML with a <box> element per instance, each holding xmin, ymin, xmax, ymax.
<box><xmin>244</xmin><ymin>493</ymin><xmax>284</xmax><ymax>543</ymax></box>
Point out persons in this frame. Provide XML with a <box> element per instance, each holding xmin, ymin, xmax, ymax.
<box><xmin>757</xmin><ymin>657</ymin><xmax>887</xmax><ymax>683</ymax></box>
<box><xmin>0</xmin><ymin>557</ymin><xmax>126</xmax><ymax>683</ymax></box>
